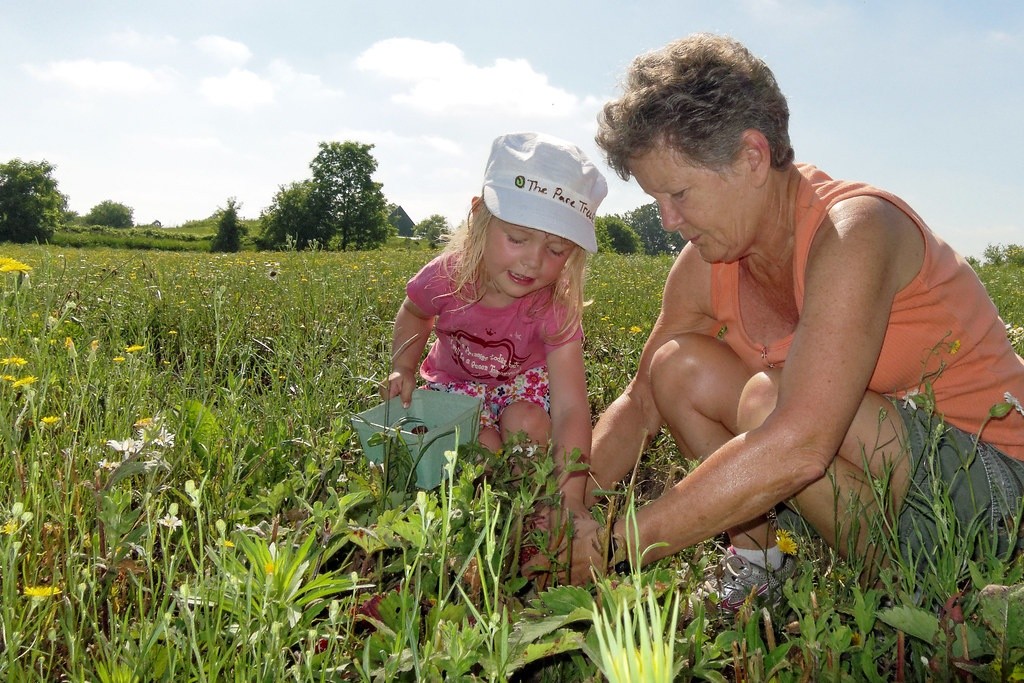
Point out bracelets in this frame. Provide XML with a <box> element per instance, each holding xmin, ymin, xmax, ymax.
<box><xmin>596</xmin><ymin>522</ymin><xmax>635</xmax><ymax>577</ymax></box>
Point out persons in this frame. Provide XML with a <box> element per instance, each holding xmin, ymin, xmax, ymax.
<box><xmin>378</xmin><ymin>132</ymin><xmax>609</xmax><ymax>552</ymax></box>
<box><xmin>520</xmin><ymin>31</ymin><xmax>1024</xmax><ymax>620</ymax></box>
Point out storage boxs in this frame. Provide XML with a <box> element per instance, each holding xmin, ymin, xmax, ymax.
<box><xmin>350</xmin><ymin>388</ymin><xmax>485</xmax><ymax>491</ymax></box>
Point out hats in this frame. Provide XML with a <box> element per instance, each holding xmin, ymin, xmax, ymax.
<box><xmin>480</xmin><ymin>132</ymin><xmax>609</xmax><ymax>254</ymax></box>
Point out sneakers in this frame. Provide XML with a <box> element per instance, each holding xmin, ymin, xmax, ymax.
<box><xmin>672</xmin><ymin>544</ymin><xmax>801</xmax><ymax>626</ymax></box>
